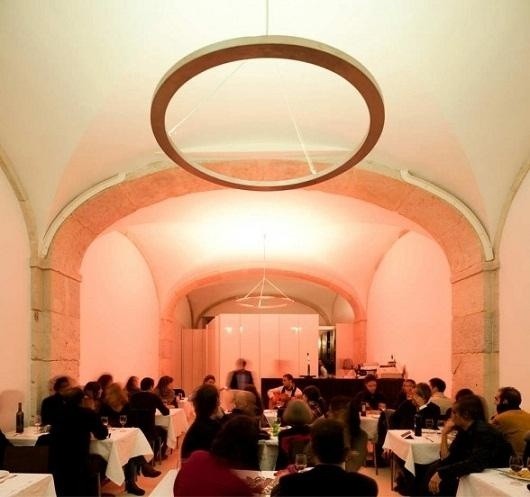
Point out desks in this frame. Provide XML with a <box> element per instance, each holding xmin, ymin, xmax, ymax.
<box><xmin>223</xmin><ymin>408</ymin><xmax>278</xmax><ymax>427</ymax></box>
<box><xmin>359</xmin><ymin>409</ymin><xmax>399</xmax><ymax>476</ymax></box>
<box><xmin>155</xmin><ymin>408</ymin><xmax>188</xmax><ymax>452</ymax></box>
<box><xmin>147</xmin><ymin>467</ymin><xmax>313</xmax><ymax>496</ymax></box>
<box><xmin>257</xmin><ymin>426</ymin><xmax>293</xmax><ymax>470</ymax></box>
<box><xmin>455</xmin><ymin>466</ymin><xmax>530</xmax><ymax>496</ymax></box>
<box><xmin>176</xmin><ymin>395</ymin><xmax>193</xmax><ymax>419</ymax></box>
<box><xmin>0</xmin><ymin>423</ymin><xmax>155</xmax><ymax>497</ymax></box>
<box><xmin>381</xmin><ymin>427</ymin><xmax>459</xmax><ymax>491</ymax></box>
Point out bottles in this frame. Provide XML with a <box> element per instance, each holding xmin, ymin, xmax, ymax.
<box><xmin>413</xmin><ymin>406</ymin><xmax>423</xmax><ymax>436</ymax></box>
<box><xmin>15</xmin><ymin>402</ymin><xmax>24</xmax><ymax>433</ymax></box>
<box><xmin>271</xmin><ymin>422</ymin><xmax>278</xmax><ymax>436</ymax></box>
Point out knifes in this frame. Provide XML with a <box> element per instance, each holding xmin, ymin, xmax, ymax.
<box><xmin>425</xmin><ymin>436</ymin><xmax>433</xmax><ymax>442</ymax></box>
<box><xmin>0</xmin><ymin>473</ymin><xmax>16</xmax><ymax>483</ymax></box>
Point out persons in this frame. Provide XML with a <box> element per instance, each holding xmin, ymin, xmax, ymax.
<box><xmin>319</xmin><ymin>359</ymin><xmax>328</xmax><ymax>378</ymax></box>
<box><xmin>353</xmin><ymin>374</ymin><xmax>388</xmax><ymax>468</ymax></box>
<box><xmin>271</xmin><ymin>419</ymin><xmax>378</xmax><ymax>496</ymax></box>
<box><xmin>1</xmin><ymin>374</ymin><xmax>179</xmax><ymax>496</ymax></box>
<box><xmin>180</xmin><ymin>358</ymin><xmax>271</xmax><ymax>471</ymax></box>
<box><xmin>391</xmin><ymin>378</ymin><xmax>529</xmax><ymax>496</ymax></box>
<box><xmin>268</xmin><ymin>374</ymin><xmax>361</xmax><ymax>473</ymax></box>
<box><xmin>174</xmin><ymin>416</ymin><xmax>264</xmax><ymax>496</ymax></box>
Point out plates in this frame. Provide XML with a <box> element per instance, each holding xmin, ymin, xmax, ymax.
<box><xmin>0</xmin><ymin>470</ymin><xmax>10</xmax><ymax>478</ymax></box>
<box><xmin>503</xmin><ymin>469</ymin><xmax>530</xmax><ymax>480</ymax></box>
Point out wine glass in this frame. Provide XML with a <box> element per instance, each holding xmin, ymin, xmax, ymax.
<box><xmin>33</xmin><ymin>415</ymin><xmax>42</xmax><ymax>436</ymax></box>
<box><xmin>119</xmin><ymin>415</ymin><xmax>128</xmax><ymax>433</ymax></box>
<box><xmin>508</xmin><ymin>456</ymin><xmax>525</xmax><ymax>488</ymax></box>
<box><xmin>425</xmin><ymin>419</ymin><xmax>433</xmax><ymax>440</ymax></box>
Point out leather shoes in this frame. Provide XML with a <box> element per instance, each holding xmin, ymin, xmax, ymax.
<box><xmin>126</xmin><ymin>481</ymin><xmax>145</xmax><ymax>495</ymax></box>
<box><xmin>141</xmin><ymin>469</ymin><xmax>161</xmax><ymax>477</ymax></box>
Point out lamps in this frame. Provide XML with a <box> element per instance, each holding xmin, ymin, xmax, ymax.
<box><xmin>146</xmin><ymin>0</ymin><xmax>387</xmax><ymax>195</ymax></box>
<box><xmin>235</xmin><ymin>231</ymin><xmax>295</xmax><ymax>309</ymax></box>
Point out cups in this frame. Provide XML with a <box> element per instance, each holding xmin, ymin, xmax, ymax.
<box><xmin>361</xmin><ymin>402</ymin><xmax>366</xmax><ymax>416</ymax></box>
<box><xmin>378</xmin><ymin>402</ymin><xmax>387</xmax><ymax>416</ymax></box>
<box><xmin>275</xmin><ymin>418</ymin><xmax>281</xmax><ymax>427</ymax></box>
<box><xmin>527</xmin><ymin>457</ymin><xmax>530</xmax><ymax>470</ymax></box>
<box><xmin>437</xmin><ymin>419</ymin><xmax>444</xmax><ymax>430</ymax></box>
<box><xmin>295</xmin><ymin>453</ymin><xmax>307</xmax><ymax>472</ymax></box>
<box><xmin>100</xmin><ymin>417</ymin><xmax>108</xmax><ymax>426</ymax></box>
